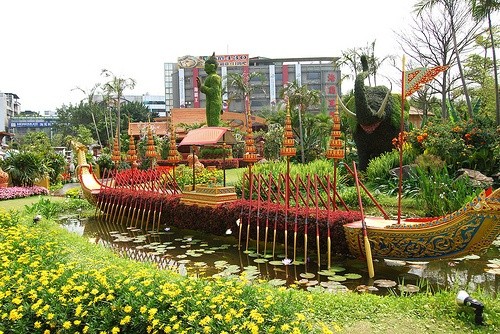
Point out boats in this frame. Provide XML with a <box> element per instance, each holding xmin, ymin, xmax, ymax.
<box><xmin>68</xmin><ymin>80</ymin><xmax>499</xmax><ymax>275</ymax></box>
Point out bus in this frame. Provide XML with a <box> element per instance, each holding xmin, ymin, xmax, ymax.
<box><xmin>46</xmin><ymin>147</ymin><xmax>73</xmax><ymax>162</ymax></box>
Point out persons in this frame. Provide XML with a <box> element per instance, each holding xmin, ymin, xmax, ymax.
<box><xmin>197</xmin><ymin>52</ymin><xmax>224</xmax><ymax>127</ymax></box>
